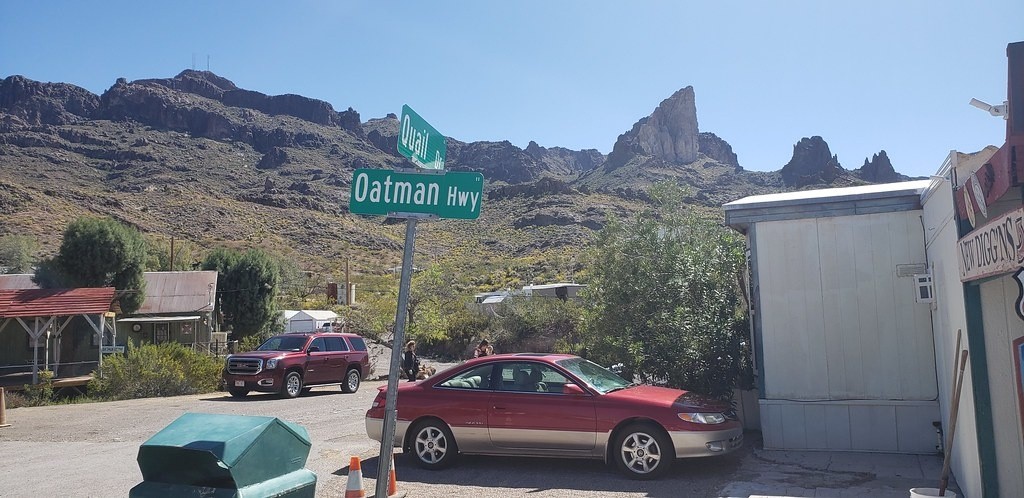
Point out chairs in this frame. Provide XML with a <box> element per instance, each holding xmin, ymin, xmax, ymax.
<box><xmin>530</xmin><ymin>370</ymin><xmax>549</xmax><ymax>393</ymax></box>
<box><xmin>515</xmin><ymin>371</ymin><xmax>531</xmax><ymax>391</ymax></box>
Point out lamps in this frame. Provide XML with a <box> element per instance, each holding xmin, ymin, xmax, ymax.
<box><xmin>968</xmin><ymin>98</ymin><xmax>1009</xmax><ymax>122</ymax></box>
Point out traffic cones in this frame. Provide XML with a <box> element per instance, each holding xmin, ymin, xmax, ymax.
<box><xmin>386</xmin><ymin>454</ymin><xmax>407</xmax><ymax>498</ymax></box>
<box><xmin>0</xmin><ymin>387</ymin><xmax>12</xmax><ymax>428</ymax></box>
<box><xmin>344</xmin><ymin>456</ymin><xmax>365</xmax><ymax>498</ymax></box>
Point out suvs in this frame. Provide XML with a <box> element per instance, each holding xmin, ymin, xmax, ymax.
<box><xmin>222</xmin><ymin>331</ymin><xmax>372</xmax><ymax>398</ymax></box>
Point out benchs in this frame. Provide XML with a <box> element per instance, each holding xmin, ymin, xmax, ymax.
<box><xmin>445</xmin><ymin>375</ymin><xmax>489</xmax><ymax>388</ymax></box>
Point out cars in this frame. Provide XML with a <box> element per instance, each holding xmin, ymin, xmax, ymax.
<box><xmin>365</xmin><ymin>352</ymin><xmax>744</xmax><ymax>482</ymax></box>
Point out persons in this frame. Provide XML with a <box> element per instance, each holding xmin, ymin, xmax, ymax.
<box><xmin>474</xmin><ymin>340</ymin><xmax>496</xmax><ymax>358</ymax></box>
<box><xmin>404</xmin><ymin>340</ymin><xmax>420</xmax><ymax>381</ymax></box>
